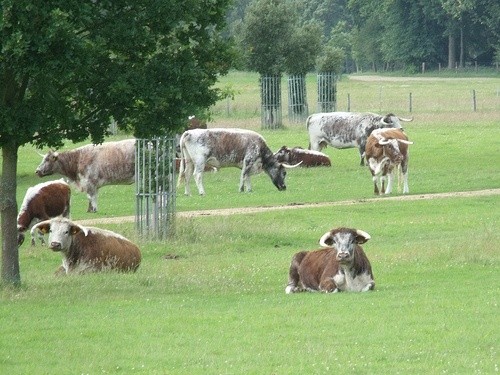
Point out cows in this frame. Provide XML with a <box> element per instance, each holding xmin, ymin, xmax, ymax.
<box><xmin>305</xmin><ymin>113</ymin><xmax>415</xmax><ymax>166</ymax></box>
<box><xmin>35</xmin><ymin>139</ymin><xmax>135</xmax><ymax>213</ymax></box>
<box><xmin>17</xmin><ymin>178</ymin><xmax>72</xmax><ymax>248</ymax></box>
<box><xmin>29</xmin><ymin>216</ymin><xmax>142</xmax><ymax>276</ymax></box>
<box><xmin>271</xmin><ymin>146</ymin><xmax>333</xmax><ymax>168</ymax></box>
<box><xmin>286</xmin><ymin>227</ymin><xmax>374</xmax><ymax>294</ymax></box>
<box><xmin>177</xmin><ymin>128</ymin><xmax>303</xmax><ymax>196</ymax></box>
<box><xmin>364</xmin><ymin>128</ymin><xmax>412</xmax><ymax>195</ymax></box>
